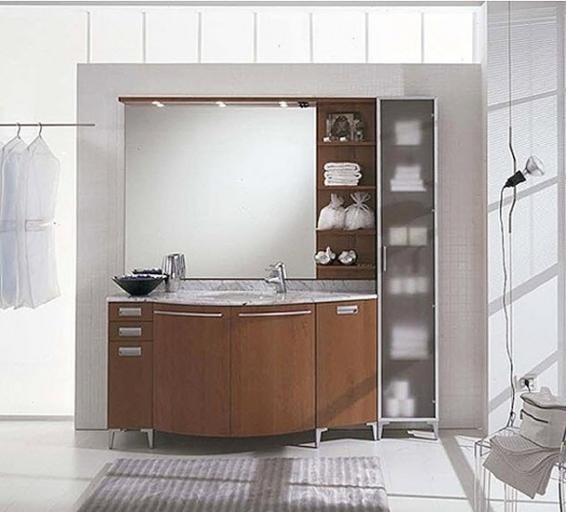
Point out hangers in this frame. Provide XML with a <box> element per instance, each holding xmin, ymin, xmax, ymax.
<box><xmin>3</xmin><ymin>123</ymin><xmax>27</xmax><ymax>150</ymax></box>
<box><xmin>23</xmin><ymin>123</ymin><xmax>51</xmax><ymax>153</ymax></box>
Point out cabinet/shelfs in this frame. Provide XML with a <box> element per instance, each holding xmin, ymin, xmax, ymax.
<box><xmin>118</xmin><ymin>96</ymin><xmax>377</xmax><ymax>280</ymax></box>
<box><xmin>377</xmin><ymin>96</ymin><xmax>439</xmax><ymax>440</ymax></box>
<box><xmin>108</xmin><ymin>300</ymin><xmax>377</xmax><ymax>448</ymax></box>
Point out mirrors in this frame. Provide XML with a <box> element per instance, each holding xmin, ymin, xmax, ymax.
<box><xmin>124</xmin><ymin>102</ymin><xmax>317</xmax><ymax>279</ymax></box>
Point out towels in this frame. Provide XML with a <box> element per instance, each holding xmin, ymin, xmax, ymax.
<box><xmin>481</xmin><ymin>434</ymin><xmax>560</xmax><ymax>500</ymax></box>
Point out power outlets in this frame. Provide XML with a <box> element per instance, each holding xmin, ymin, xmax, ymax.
<box><xmin>514</xmin><ymin>375</ymin><xmax>536</xmax><ymax>392</ymax></box>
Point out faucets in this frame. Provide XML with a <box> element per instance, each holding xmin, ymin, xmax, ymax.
<box><xmin>265</xmin><ymin>262</ymin><xmax>288</xmax><ymax>295</ymax></box>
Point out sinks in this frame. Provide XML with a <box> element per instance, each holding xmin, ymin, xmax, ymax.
<box><xmin>192</xmin><ymin>291</ymin><xmax>286</xmax><ymax>303</ymax></box>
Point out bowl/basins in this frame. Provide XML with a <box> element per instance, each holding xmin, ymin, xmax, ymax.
<box><xmin>129</xmin><ymin>268</ymin><xmax>162</xmax><ymax>275</ymax></box>
<box><xmin>109</xmin><ymin>273</ymin><xmax>167</xmax><ymax>296</ymax></box>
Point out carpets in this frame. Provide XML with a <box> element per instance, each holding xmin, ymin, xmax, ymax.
<box><xmin>77</xmin><ymin>455</ymin><xmax>386</xmax><ymax>511</ymax></box>
